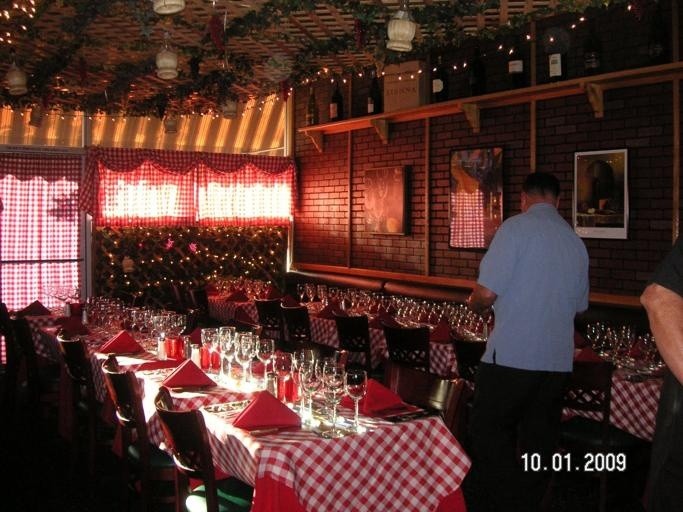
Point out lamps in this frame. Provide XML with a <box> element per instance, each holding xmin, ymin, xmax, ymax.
<box><xmin>379</xmin><ymin>0</ymin><xmax>418</xmax><ymax>53</ymax></box>
<box><xmin>154</xmin><ymin>1</ymin><xmax>178</xmax><ymax>82</ymax></box>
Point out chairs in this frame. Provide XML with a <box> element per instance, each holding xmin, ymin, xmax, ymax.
<box><xmin>2</xmin><ymin>275</ymin><xmax>660</xmax><ymax>510</ymax></box>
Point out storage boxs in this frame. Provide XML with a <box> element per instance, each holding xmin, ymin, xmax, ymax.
<box><xmin>383</xmin><ymin>59</ymin><xmax>429</xmax><ymax>112</ymax></box>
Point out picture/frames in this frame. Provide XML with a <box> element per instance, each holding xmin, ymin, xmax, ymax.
<box><xmin>447</xmin><ymin>146</ymin><xmax>505</xmax><ymax>252</ymax></box>
<box><xmin>364</xmin><ymin>164</ymin><xmax>408</xmax><ymax>237</ymax></box>
<box><xmin>573</xmin><ymin>150</ymin><xmax>627</xmax><ymax>240</ymax></box>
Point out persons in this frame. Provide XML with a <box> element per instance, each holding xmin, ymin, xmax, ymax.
<box><xmin>639</xmin><ymin>232</ymin><xmax>683</xmax><ymax>511</ymax></box>
<box><xmin>464</xmin><ymin>171</ymin><xmax>590</xmax><ymax>509</ymax></box>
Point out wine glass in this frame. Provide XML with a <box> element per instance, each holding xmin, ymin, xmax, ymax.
<box><xmin>42</xmin><ymin>284</ymin><xmax>79</xmax><ymax>313</ymax></box>
<box><xmin>199</xmin><ymin>324</ymin><xmax>370</xmax><ymax>441</ymax></box>
<box><xmin>294</xmin><ymin>281</ymin><xmax>661</xmax><ymax>375</ymax></box>
<box><xmin>209</xmin><ymin>277</ymin><xmax>304</xmax><ymax>309</ymax></box>
<box><xmin>84</xmin><ymin>294</ymin><xmax>199</xmax><ymax>368</ymax></box>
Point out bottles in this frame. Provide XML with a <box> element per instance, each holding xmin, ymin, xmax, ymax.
<box><xmin>306</xmin><ymin>13</ymin><xmax>665</xmax><ymax>127</ymax></box>
